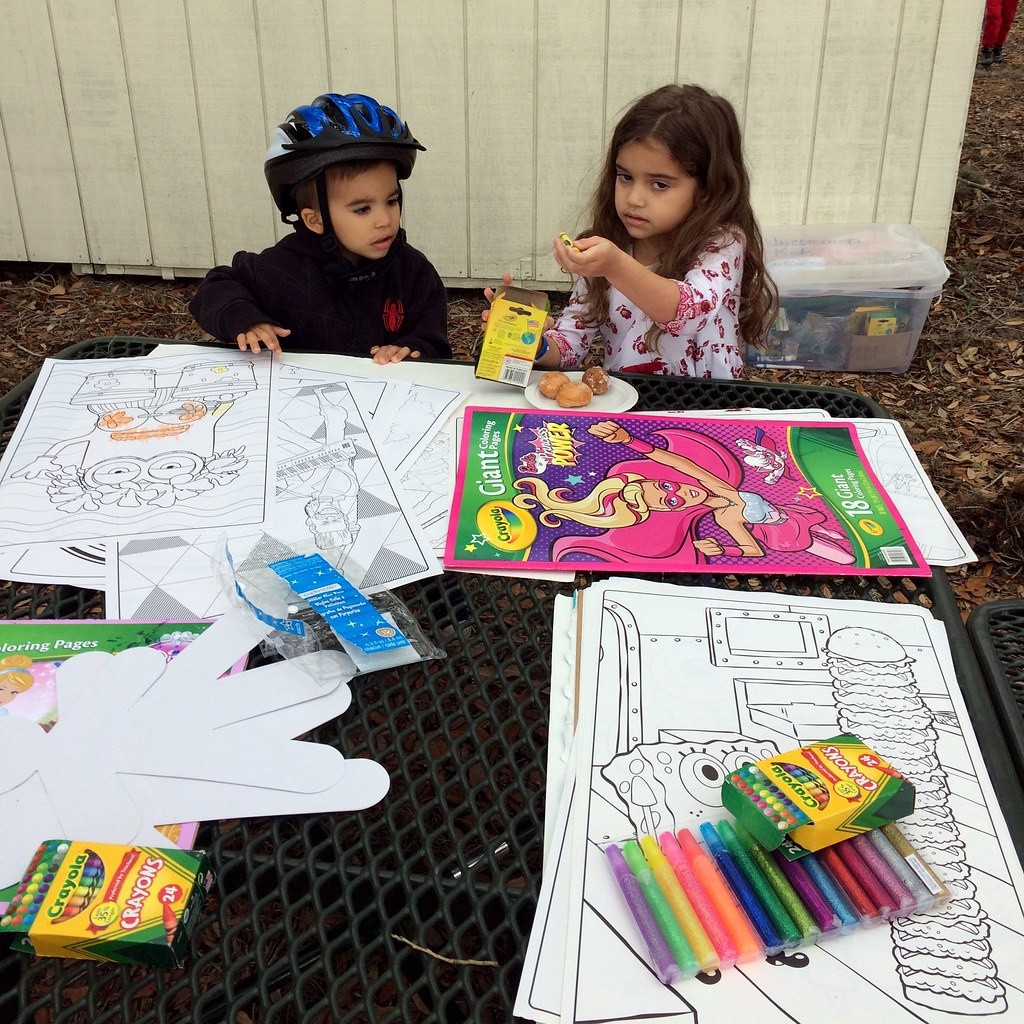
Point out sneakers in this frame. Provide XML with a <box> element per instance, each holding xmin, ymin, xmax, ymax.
<box><xmin>980</xmin><ymin>44</ymin><xmax>1003</xmax><ymax>65</ymax></box>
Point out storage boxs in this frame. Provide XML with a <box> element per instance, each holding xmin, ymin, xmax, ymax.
<box><xmin>725</xmin><ymin>730</ymin><xmax>916</xmax><ymax>861</ymax></box>
<box><xmin>474</xmin><ymin>284</ymin><xmax>549</xmax><ymax>391</ymax></box>
<box><xmin>0</xmin><ymin>839</ymin><xmax>216</xmax><ymax>967</ymax></box>
<box><xmin>762</xmin><ymin>219</ymin><xmax>951</xmax><ymax>374</ymax></box>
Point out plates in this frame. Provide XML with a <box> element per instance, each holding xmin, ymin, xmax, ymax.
<box><xmin>525</xmin><ymin>372</ymin><xmax>638</xmax><ymax>412</ymax></box>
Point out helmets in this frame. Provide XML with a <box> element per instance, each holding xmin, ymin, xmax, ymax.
<box><xmin>263</xmin><ymin>93</ymin><xmax>427</xmax><ymax>224</ymax></box>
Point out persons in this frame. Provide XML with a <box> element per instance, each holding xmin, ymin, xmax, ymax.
<box><xmin>482</xmin><ymin>84</ymin><xmax>780</xmax><ymax>381</ymax></box>
<box><xmin>188</xmin><ymin>93</ymin><xmax>454</xmax><ymax>364</ymax></box>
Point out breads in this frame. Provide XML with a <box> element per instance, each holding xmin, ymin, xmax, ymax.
<box><xmin>556</xmin><ymin>382</ymin><xmax>593</xmax><ymax>406</ymax></box>
<box><xmin>539</xmin><ymin>371</ymin><xmax>570</xmax><ymax>399</ymax></box>
<box><xmin>582</xmin><ymin>366</ymin><xmax>610</xmax><ymax>395</ymax></box>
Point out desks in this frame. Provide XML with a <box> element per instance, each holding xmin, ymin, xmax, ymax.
<box><xmin>0</xmin><ymin>334</ymin><xmax>1024</xmax><ymax>1024</ymax></box>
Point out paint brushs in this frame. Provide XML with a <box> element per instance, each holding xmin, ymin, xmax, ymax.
<box><xmin>605</xmin><ymin>819</ymin><xmax>950</xmax><ymax>983</ymax></box>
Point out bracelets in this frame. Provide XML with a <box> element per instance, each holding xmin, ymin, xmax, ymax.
<box><xmin>535</xmin><ymin>337</ymin><xmax>548</xmax><ymax>362</ymax></box>
